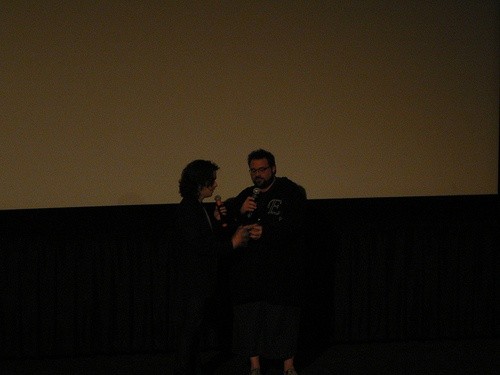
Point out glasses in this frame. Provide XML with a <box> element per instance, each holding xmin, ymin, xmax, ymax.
<box><xmin>248</xmin><ymin>166</ymin><xmax>270</xmax><ymax>174</ymax></box>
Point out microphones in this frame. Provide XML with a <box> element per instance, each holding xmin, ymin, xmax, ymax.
<box><xmin>215</xmin><ymin>195</ymin><xmax>228</xmax><ymax>228</ymax></box>
<box><xmin>248</xmin><ymin>188</ymin><xmax>262</xmax><ymax>217</ymax></box>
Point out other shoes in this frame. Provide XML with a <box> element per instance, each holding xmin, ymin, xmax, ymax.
<box><xmin>283</xmin><ymin>367</ymin><xmax>298</xmax><ymax>375</ymax></box>
<box><xmin>248</xmin><ymin>368</ymin><xmax>262</xmax><ymax>375</ymax></box>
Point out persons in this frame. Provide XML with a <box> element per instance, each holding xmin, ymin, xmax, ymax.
<box><xmin>169</xmin><ymin>159</ymin><xmax>248</xmax><ymax>375</ymax></box>
<box><xmin>234</xmin><ymin>149</ymin><xmax>311</xmax><ymax>375</ymax></box>
